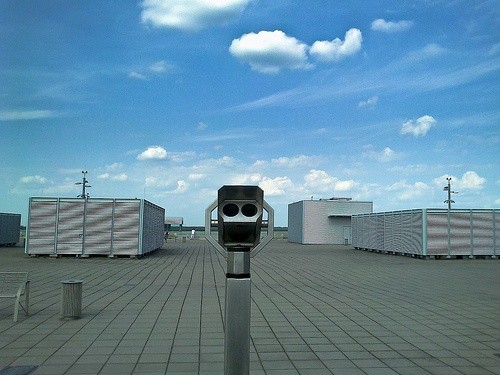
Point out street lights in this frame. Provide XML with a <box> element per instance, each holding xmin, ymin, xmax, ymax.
<box><xmin>74</xmin><ymin>171</ymin><xmax>92</xmax><ymax>258</ymax></box>
<box><xmin>444</xmin><ymin>177</ymin><xmax>458</xmax><ymax>259</ymax></box>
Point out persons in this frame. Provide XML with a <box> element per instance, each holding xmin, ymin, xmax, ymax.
<box><xmin>164</xmin><ymin>231</ymin><xmax>170</xmax><ymax>243</ymax></box>
<box><xmin>191</xmin><ymin>229</ymin><xmax>196</xmax><ymax>239</ymax></box>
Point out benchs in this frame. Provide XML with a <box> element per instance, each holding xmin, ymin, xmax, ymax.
<box><xmin>0</xmin><ymin>272</ymin><xmax>30</xmax><ymax>322</ymax></box>
<box><xmin>164</xmin><ymin>235</ymin><xmax>176</xmax><ymax>243</ymax></box>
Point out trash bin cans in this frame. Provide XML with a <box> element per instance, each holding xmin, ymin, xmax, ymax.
<box><xmin>59</xmin><ymin>278</ymin><xmax>84</xmax><ymax>321</ymax></box>
